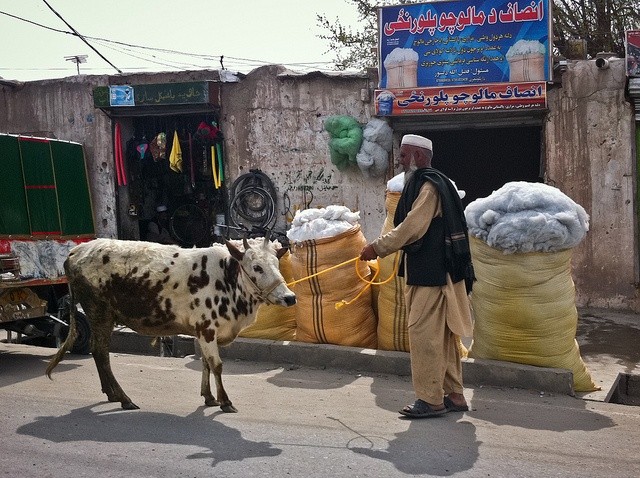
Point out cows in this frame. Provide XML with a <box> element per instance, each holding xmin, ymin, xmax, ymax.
<box><xmin>45</xmin><ymin>227</ymin><xmax>300</xmax><ymax>417</ymax></box>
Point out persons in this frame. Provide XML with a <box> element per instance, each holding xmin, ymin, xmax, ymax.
<box><xmin>359</xmin><ymin>134</ymin><xmax>477</xmax><ymax>418</ymax></box>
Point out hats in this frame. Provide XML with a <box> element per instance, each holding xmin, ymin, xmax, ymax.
<box><xmin>402</xmin><ymin>133</ymin><xmax>432</xmax><ymax>152</ymax></box>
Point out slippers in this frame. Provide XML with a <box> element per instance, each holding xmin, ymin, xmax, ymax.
<box><xmin>443</xmin><ymin>394</ymin><xmax>468</xmax><ymax>411</ymax></box>
<box><xmin>397</xmin><ymin>398</ymin><xmax>448</xmax><ymax>416</ymax></box>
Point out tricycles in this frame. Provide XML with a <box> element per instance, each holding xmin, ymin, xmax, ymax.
<box><xmin>0</xmin><ymin>131</ymin><xmax>98</xmax><ymax>354</ymax></box>
